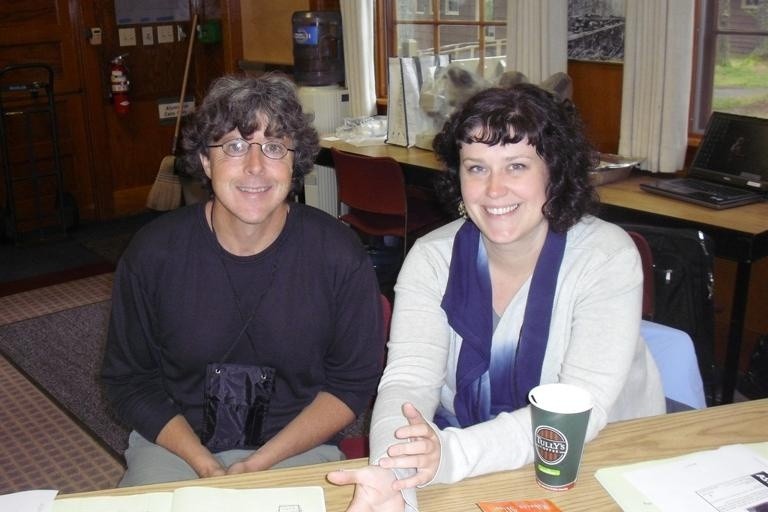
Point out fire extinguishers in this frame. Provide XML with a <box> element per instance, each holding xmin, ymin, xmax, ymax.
<box><xmin>109</xmin><ymin>52</ymin><xmax>132</xmax><ymax>115</ymax></box>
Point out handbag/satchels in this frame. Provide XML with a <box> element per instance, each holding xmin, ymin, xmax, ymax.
<box><xmin>383</xmin><ymin>53</ymin><xmax>452</xmax><ymax>149</ymax></box>
<box><xmin>200</xmin><ymin>361</ymin><xmax>276</xmax><ymax>454</ymax></box>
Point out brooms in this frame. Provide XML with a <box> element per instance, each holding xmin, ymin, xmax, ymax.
<box><xmin>145</xmin><ymin>15</ymin><xmax>198</xmax><ymax>212</ymax></box>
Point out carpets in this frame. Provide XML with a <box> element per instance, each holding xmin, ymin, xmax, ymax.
<box><xmin>1</xmin><ymin>204</ymin><xmax>174</xmax><ymax>298</ymax></box>
<box><xmin>1</xmin><ymin>298</ymin><xmax>146</xmax><ymax>469</ymax></box>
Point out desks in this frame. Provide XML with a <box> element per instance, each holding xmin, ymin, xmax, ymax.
<box><xmin>313</xmin><ymin>128</ymin><xmax>767</xmax><ymax>408</ymax></box>
<box><xmin>57</xmin><ymin>396</ymin><xmax>764</xmax><ymax>512</ymax></box>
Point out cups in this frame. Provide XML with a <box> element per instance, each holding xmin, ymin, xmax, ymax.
<box><xmin>528</xmin><ymin>380</ymin><xmax>599</xmax><ymax>493</ymax></box>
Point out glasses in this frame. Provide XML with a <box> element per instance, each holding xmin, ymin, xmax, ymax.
<box><xmin>206</xmin><ymin>139</ymin><xmax>296</xmax><ymax>159</ymax></box>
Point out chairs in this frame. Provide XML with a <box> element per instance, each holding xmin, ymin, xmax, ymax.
<box><xmin>329</xmin><ymin>144</ymin><xmax>447</xmax><ymax>264</ymax></box>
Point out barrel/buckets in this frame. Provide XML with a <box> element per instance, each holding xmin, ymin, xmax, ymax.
<box><xmin>291</xmin><ymin>10</ymin><xmax>342</xmax><ymax>87</ymax></box>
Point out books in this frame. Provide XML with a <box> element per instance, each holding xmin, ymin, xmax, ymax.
<box><xmin>594</xmin><ymin>443</ymin><xmax>768</xmax><ymax>512</ymax></box>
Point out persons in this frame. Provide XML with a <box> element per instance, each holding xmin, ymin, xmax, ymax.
<box><xmin>325</xmin><ymin>84</ymin><xmax>665</xmax><ymax>512</ymax></box>
<box><xmin>101</xmin><ymin>70</ymin><xmax>385</xmax><ymax>487</ymax></box>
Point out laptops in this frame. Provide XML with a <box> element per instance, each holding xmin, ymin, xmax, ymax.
<box><xmin>639</xmin><ymin>111</ymin><xmax>768</xmax><ymax>210</ymax></box>
